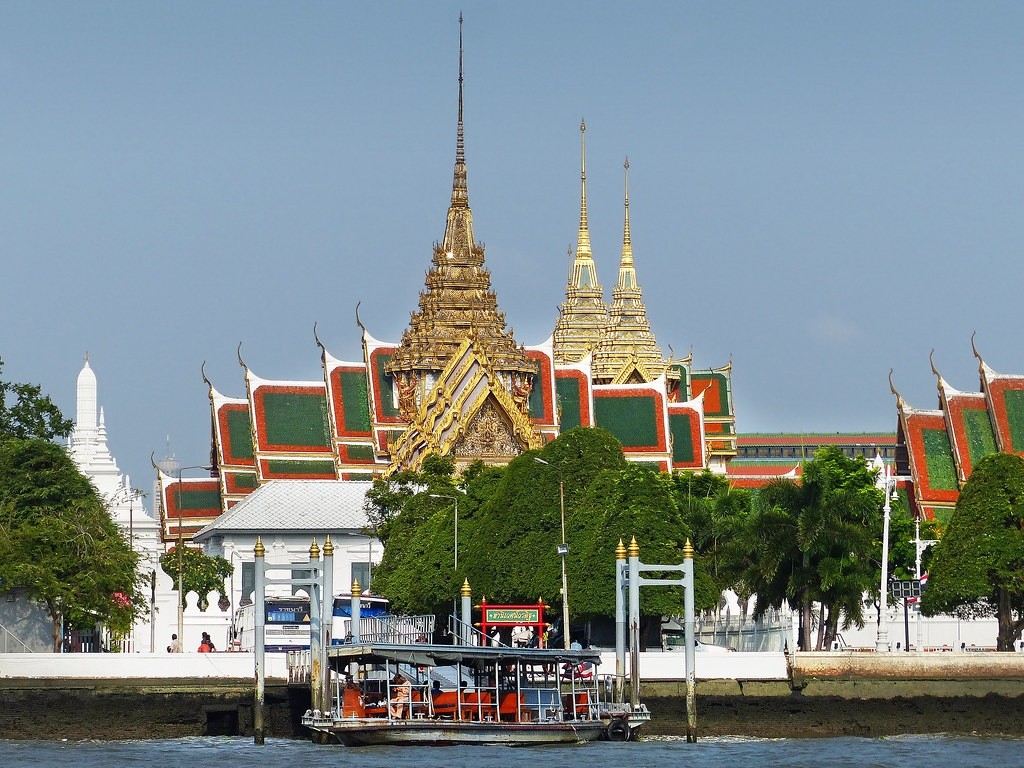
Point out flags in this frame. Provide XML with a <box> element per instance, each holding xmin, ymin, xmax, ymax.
<box><xmin>562</xmin><ymin>661</ymin><xmax>592</xmax><ymax>679</ymax></box>
<box><xmin>907</xmin><ymin>574</ymin><xmax>927</xmax><ymax>603</ymax></box>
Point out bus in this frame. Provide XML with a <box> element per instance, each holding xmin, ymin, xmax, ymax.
<box><xmin>332</xmin><ymin>595</ymin><xmax>389</xmax><ymax>646</ymax></box>
<box><xmin>232</xmin><ymin>596</ymin><xmax>311</xmax><ymax>652</ymax></box>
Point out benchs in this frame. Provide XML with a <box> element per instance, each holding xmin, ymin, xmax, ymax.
<box><xmin>342</xmin><ymin>688</ymin><xmax>562</xmax><ymax>723</ymax></box>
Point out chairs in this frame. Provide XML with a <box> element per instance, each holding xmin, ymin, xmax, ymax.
<box><xmin>567</xmin><ymin>694</ymin><xmax>589</xmax><ymax>720</ymax></box>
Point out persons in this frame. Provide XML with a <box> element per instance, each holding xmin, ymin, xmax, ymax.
<box><xmin>169</xmin><ymin>633</ymin><xmax>183</xmax><ymax>653</ymax></box>
<box><xmin>387</xmin><ymin>674</ymin><xmax>501</xmax><ymax>720</ymax></box>
<box><xmin>197</xmin><ymin>631</ymin><xmax>217</xmax><ymax>653</ymax></box>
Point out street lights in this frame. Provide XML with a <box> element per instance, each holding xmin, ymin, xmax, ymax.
<box><xmin>177</xmin><ymin>464</ymin><xmax>214</xmax><ymax>652</ymax></box>
<box><xmin>129</xmin><ymin>492</ymin><xmax>149</xmax><ymax>553</ymax></box>
<box><xmin>534</xmin><ymin>457</ymin><xmax>571</xmax><ymax>649</ymax></box>
<box><xmin>430</xmin><ymin>494</ymin><xmax>458</xmax><ymax>646</ymax></box>
<box><xmin>348</xmin><ymin>533</ymin><xmax>371</xmax><ymax>596</ymax></box>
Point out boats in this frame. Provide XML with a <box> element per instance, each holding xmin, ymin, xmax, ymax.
<box><xmin>300</xmin><ymin>641</ymin><xmax>651</xmax><ymax>747</ymax></box>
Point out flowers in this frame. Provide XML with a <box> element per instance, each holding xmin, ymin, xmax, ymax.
<box><xmin>111</xmin><ymin>592</ymin><xmax>134</xmax><ymax>608</ymax></box>
